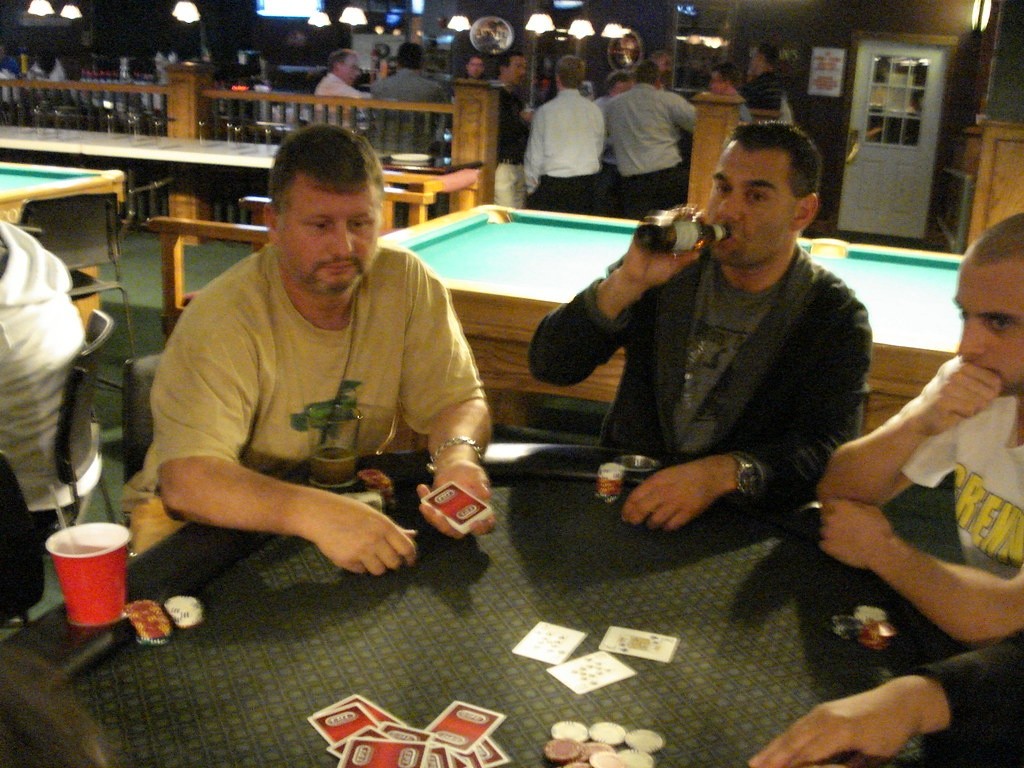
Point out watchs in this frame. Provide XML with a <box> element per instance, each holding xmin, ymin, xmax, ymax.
<box><xmin>732</xmin><ymin>455</ymin><xmax>758</xmax><ymax>498</ymax></box>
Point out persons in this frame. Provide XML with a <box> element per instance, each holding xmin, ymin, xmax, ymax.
<box><xmin>815</xmin><ymin>212</ymin><xmax>1024</xmax><ymax>650</ymax></box>
<box><xmin>141</xmin><ymin>122</ymin><xmax>495</xmax><ymax>578</ymax></box>
<box><xmin>750</xmin><ymin>641</ymin><xmax>1024</xmax><ymax>768</ymax></box>
<box><xmin>262</xmin><ymin>30</ymin><xmax>785</xmax><ymax>218</ymax></box>
<box><xmin>528</xmin><ymin>117</ymin><xmax>874</xmax><ymax>533</ymax></box>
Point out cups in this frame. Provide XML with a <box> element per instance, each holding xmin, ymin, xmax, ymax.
<box><xmin>45</xmin><ymin>521</ymin><xmax>132</xmax><ymax>627</ymax></box>
<box><xmin>304</xmin><ymin>403</ymin><xmax>361</xmax><ymax>489</ymax></box>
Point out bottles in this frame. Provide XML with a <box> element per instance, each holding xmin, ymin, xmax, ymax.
<box><xmin>633</xmin><ymin>222</ymin><xmax>731</xmax><ymax>254</ymax></box>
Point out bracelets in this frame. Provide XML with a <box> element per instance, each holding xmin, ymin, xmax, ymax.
<box><xmin>425</xmin><ymin>437</ymin><xmax>483</xmax><ymax>476</ymax></box>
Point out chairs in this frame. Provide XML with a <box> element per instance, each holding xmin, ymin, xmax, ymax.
<box><xmin>18</xmin><ymin>191</ymin><xmax>132</xmax><ymax>397</ymax></box>
<box><xmin>385</xmin><ymin>163</ymin><xmax>482</xmax><ymax>228</ymax></box>
<box><xmin>36</xmin><ymin>306</ymin><xmax>119</xmax><ymax>538</ymax></box>
<box><xmin>0</xmin><ymin>454</ymin><xmax>44</xmax><ymax>628</ymax></box>
<box><xmin>142</xmin><ymin>193</ymin><xmax>273</xmax><ymax>337</ymax></box>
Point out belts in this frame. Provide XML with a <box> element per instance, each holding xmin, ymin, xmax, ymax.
<box><xmin>498</xmin><ymin>158</ymin><xmax>524</xmax><ymax>165</ymax></box>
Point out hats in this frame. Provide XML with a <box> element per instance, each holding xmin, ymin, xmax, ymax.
<box><xmin>390</xmin><ymin>44</ymin><xmax>425</xmax><ymax>67</ymax></box>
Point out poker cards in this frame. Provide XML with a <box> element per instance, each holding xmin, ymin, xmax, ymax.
<box><xmin>599</xmin><ymin>625</ymin><xmax>681</xmax><ymax>663</ymax></box>
<box><xmin>545</xmin><ymin>651</ymin><xmax>638</xmax><ymax>695</ymax></box>
<box><xmin>512</xmin><ymin>621</ymin><xmax>589</xmax><ymax>665</ymax></box>
<box><xmin>307</xmin><ymin>694</ymin><xmax>512</xmax><ymax>768</ymax></box>
<box><xmin>420</xmin><ymin>481</ymin><xmax>493</xmax><ymax>535</ymax></box>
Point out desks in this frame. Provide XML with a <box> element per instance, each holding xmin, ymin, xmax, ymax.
<box><xmin>376</xmin><ymin>204</ymin><xmax>966</xmax><ymax>441</ymax></box>
<box><xmin>0</xmin><ymin>443</ymin><xmax>922</xmax><ymax>768</ymax></box>
<box><xmin>0</xmin><ymin>125</ymin><xmax>280</xmax><ymax>169</ymax></box>
<box><xmin>0</xmin><ymin>161</ymin><xmax>133</xmax><ymax>329</ymax></box>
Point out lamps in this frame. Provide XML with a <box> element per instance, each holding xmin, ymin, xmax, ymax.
<box><xmin>309</xmin><ymin>10</ymin><xmax>331</xmax><ymax>28</ymax></box>
<box><xmin>600</xmin><ymin>19</ymin><xmax>635</xmax><ymax>45</ymax></box>
<box><xmin>373</xmin><ymin>22</ymin><xmax>385</xmax><ymax>35</ymax></box>
<box><xmin>59</xmin><ymin>3</ymin><xmax>83</xmax><ymax>19</ymax></box>
<box><xmin>567</xmin><ymin>14</ymin><xmax>596</xmax><ymax>39</ymax></box>
<box><xmin>676</xmin><ymin>27</ymin><xmax>723</xmax><ymax>49</ymax></box>
<box><xmin>447</xmin><ymin>12</ymin><xmax>471</xmax><ymax>32</ymax></box>
<box><xmin>27</xmin><ymin>0</ymin><xmax>55</xmax><ymax>16</ymax></box>
<box><xmin>526</xmin><ymin>10</ymin><xmax>556</xmax><ymax>34</ymax></box>
<box><xmin>172</xmin><ymin>0</ymin><xmax>201</xmax><ymax>24</ymax></box>
<box><xmin>339</xmin><ymin>3</ymin><xmax>369</xmax><ymax>26</ymax></box>
